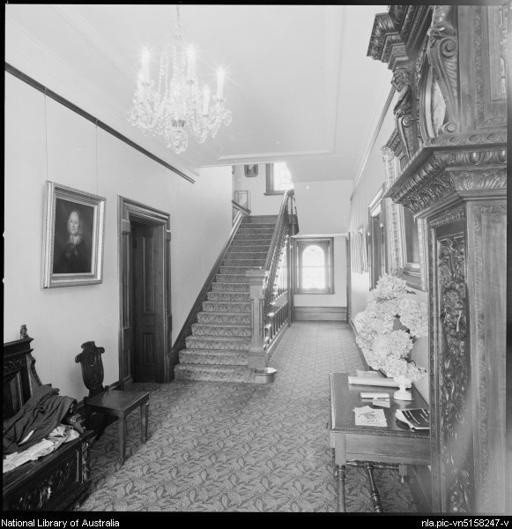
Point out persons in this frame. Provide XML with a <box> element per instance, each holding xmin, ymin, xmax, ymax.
<box><xmin>54</xmin><ymin>207</ymin><xmax>92</xmax><ymax>272</ymax></box>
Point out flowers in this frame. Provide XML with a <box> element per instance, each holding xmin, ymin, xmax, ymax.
<box><xmin>350</xmin><ymin>272</ymin><xmax>433</xmax><ymax>390</ymax></box>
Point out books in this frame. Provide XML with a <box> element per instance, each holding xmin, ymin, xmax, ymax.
<box><xmin>395</xmin><ymin>408</ymin><xmax>430</xmax><ymax>430</ymax></box>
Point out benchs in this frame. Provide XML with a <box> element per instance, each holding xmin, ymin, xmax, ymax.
<box><xmin>3</xmin><ymin>323</ymin><xmax>97</xmax><ymax>510</ymax></box>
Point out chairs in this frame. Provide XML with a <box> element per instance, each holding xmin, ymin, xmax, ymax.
<box><xmin>74</xmin><ymin>340</ymin><xmax>151</xmax><ymax>466</ymax></box>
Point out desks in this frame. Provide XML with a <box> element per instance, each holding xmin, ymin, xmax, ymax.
<box><xmin>328</xmin><ymin>367</ymin><xmax>435</xmax><ymax>512</ymax></box>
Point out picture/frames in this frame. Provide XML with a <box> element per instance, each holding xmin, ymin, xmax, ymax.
<box><xmin>42</xmin><ymin>180</ymin><xmax>108</xmax><ymax>288</ymax></box>
<box><xmin>380</xmin><ymin>124</ymin><xmax>432</xmax><ymax>293</ymax></box>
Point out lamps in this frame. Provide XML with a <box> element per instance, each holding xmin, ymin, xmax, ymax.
<box><xmin>127</xmin><ymin>5</ymin><xmax>233</xmax><ymax>158</ymax></box>
